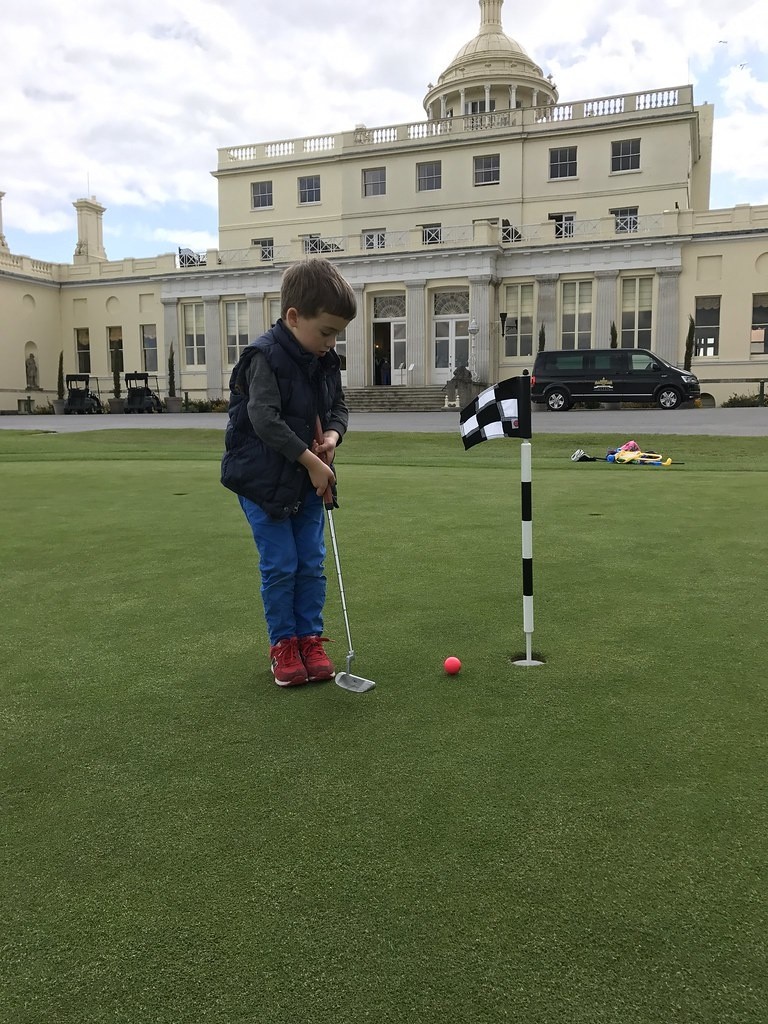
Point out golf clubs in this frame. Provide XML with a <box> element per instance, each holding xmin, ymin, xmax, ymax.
<box><xmin>313</xmin><ymin>411</ymin><xmax>377</xmax><ymax>693</ymax></box>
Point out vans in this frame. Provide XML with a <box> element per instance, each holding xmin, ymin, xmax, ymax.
<box><xmin>530</xmin><ymin>348</ymin><xmax>701</xmax><ymax>410</ymax></box>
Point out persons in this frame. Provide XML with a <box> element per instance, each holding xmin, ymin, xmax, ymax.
<box><xmin>219</xmin><ymin>257</ymin><xmax>358</xmax><ymax>689</ymax></box>
<box><xmin>374</xmin><ymin>351</ymin><xmax>391</xmax><ymax>386</ymax></box>
<box><xmin>26</xmin><ymin>353</ymin><xmax>38</xmax><ymax>388</ymax></box>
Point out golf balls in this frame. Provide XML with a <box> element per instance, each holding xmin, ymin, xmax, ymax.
<box><xmin>443</xmin><ymin>656</ymin><xmax>462</xmax><ymax>675</ymax></box>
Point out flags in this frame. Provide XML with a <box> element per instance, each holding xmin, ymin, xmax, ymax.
<box><xmin>459</xmin><ymin>374</ymin><xmax>532</xmax><ymax>452</ymax></box>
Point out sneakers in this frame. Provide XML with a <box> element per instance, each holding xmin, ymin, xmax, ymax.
<box><xmin>270</xmin><ymin>636</ymin><xmax>335</xmax><ymax>686</ymax></box>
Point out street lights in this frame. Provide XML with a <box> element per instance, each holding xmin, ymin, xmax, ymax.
<box><xmin>468</xmin><ymin>316</ymin><xmax>479</xmax><ymax>382</ymax></box>
